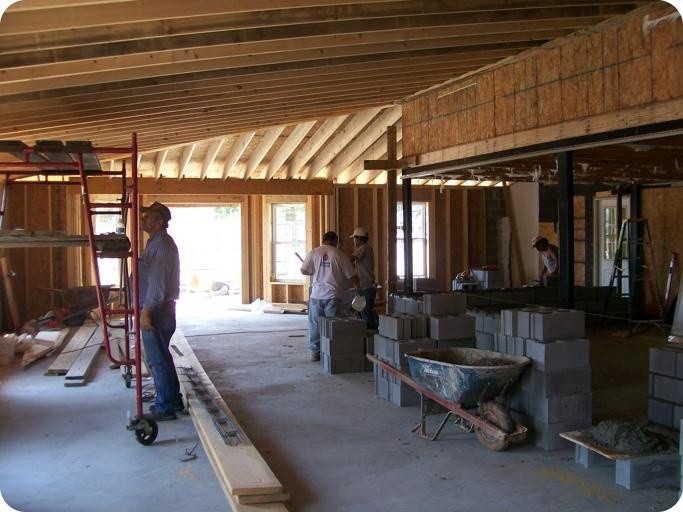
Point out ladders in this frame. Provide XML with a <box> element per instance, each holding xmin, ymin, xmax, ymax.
<box><xmin>602</xmin><ymin>217</ymin><xmax>663</xmax><ymax>337</ymax></box>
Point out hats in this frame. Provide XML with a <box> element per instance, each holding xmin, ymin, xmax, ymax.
<box><xmin>139</xmin><ymin>200</ymin><xmax>171</xmax><ymax>222</ymax></box>
<box><xmin>348</xmin><ymin>226</ymin><xmax>369</xmax><ymax>239</ymax></box>
<box><xmin>529</xmin><ymin>235</ymin><xmax>545</xmax><ymax>249</ymax></box>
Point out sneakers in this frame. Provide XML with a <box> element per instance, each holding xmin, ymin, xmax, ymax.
<box><xmin>310</xmin><ymin>351</ymin><xmax>320</xmax><ymax>361</ymax></box>
<box><xmin>143</xmin><ymin>397</ymin><xmax>185</xmax><ymax>423</ymax></box>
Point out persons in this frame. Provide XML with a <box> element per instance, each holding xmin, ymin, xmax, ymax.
<box><xmin>344</xmin><ymin>227</ymin><xmax>378</xmax><ymax>330</ymax></box>
<box><xmin>126</xmin><ymin>200</ymin><xmax>182</xmax><ymax>423</ymax></box>
<box><xmin>528</xmin><ymin>233</ymin><xmax>558</xmax><ymax>280</ymax></box>
<box><xmin>300</xmin><ymin>231</ymin><xmax>362</xmax><ymax>362</ymax></box>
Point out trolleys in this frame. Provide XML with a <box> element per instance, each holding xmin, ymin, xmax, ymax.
<box><xmin>364</xmin><ymin>345</ymin><xmax>533</xmax><ymax>453</ymax></box>
